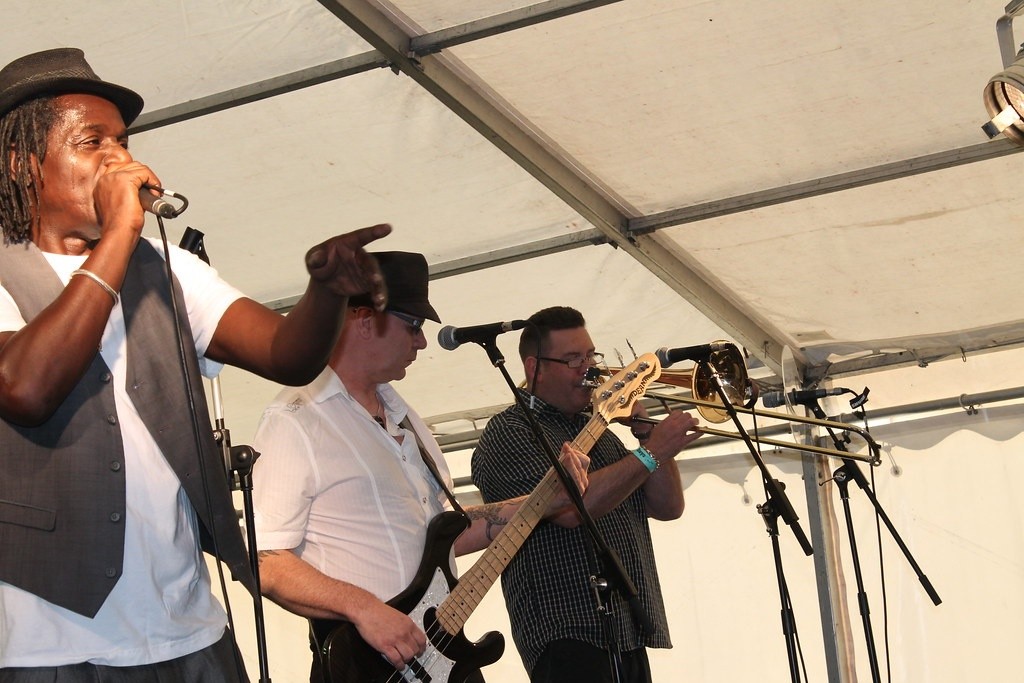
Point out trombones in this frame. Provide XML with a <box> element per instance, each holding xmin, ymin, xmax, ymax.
<box><xmin>516</xmin><ymin>340</ymin><xmax>881</xmax><ymax>466</ymax></box>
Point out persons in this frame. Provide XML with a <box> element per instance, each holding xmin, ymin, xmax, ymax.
<box><xmin>471</xmin><ymin>306</ymin><xmax>707</xmax><ymax>683</ymax></box>
<box><xmin>0</xmin><ymin>48</ymin><xmax>393</xmax><ymax>683</ymax></box>
<box><xmin>244</xmin><ymin>250</ymin><xmax>590</xmax><ymax>682</ymax></box>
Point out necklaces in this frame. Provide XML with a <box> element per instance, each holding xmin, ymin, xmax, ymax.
<box><xmin>371</xmin><ymin>392</ymin><xmax>385</xmax><ymax>430</ymax></box>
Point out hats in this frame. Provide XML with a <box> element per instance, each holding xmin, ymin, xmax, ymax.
<box><xmin>0</xmin><ymin>47</ymin><xmax>144</xmax><ymax>130</ymax></box>
<box><xmin>348</xmin><ymin>251</ymin><xmax>441</xmax><ymax>321</ymax></box>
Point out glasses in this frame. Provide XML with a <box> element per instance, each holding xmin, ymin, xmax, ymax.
<box><xmin>352</xmin><ymin>304</ymin><xmax>422</xmax><ymax>334</ymax></box>
<box><xmin>535</xmin><ymin>352</ymin><xmax>605</xmax><ymax>370</ymax></box>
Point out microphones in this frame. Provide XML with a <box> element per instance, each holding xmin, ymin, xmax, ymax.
<box><xmin>762</xmin><ymin>387</ymin><xmax>850</xmax><ymax>408</ymax></box>
<box><xmin>654</xmin><ymin>341</ymin><xmax>734</xmax><ymax>368</ymax></box>
<box><xmin>438</xmin><ymin>320</ymin><xmax>531</xmax><ymax>351</ymax></box>
<box><xmin>138</xmin><ymin>186</ymin><xmax>175</xmax><ymax>219</ymax></box>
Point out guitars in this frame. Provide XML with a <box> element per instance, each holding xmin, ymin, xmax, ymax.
<box><xmin>323</xmin><ymin>338</ymin><xmax>663</xmax><ymax>683</ymax></box>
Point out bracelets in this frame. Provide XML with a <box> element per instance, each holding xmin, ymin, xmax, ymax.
<box><xmin>73</xmin><ymin>269</ymin><xmax>119</xmax><ymax>309</ymax></box>
<box><xmin>642</xmin><ymin>445</ymin><xmax>660</xmax><ymax>469</ymax></box>
<box><xmin>631</xmin><ymin>425</ymin><xmax>656</xmax><ymax>439</ymax></box>
<box><xmin>633</xmin><ymin>447</ymin><xmax>655</xmax><ymax>473</ymax></box>
